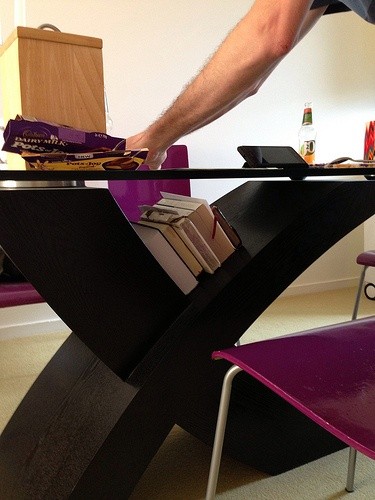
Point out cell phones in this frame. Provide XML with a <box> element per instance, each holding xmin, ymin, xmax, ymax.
<box><xmin>237</xmin><ymin>146</ymin><xmax>309</xmax><ymax>168</ymax></box>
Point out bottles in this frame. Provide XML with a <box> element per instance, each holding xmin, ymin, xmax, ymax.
<box><xmin>298</xmin><ymin>102</ymin><xmax>317</xmax><ymax>166</ymax></box>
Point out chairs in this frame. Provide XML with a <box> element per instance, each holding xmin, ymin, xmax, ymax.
<box><xmin>0</xmin><ymin>144</ymin><xmax>192</xmax><ymax>311</ymax></box>
<box><xmin>205</xmin><ymin>248</ymin><xmax>375</xmax><ymax>500</ymax></box>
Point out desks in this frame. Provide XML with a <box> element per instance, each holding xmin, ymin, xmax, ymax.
<box><xmin>0</xmin><ymin>167</ymin><xmax>375</xmax><ymax>500</ymax></box>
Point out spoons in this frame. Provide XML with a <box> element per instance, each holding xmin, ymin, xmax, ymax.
<box><xmin>330</xmin><ymin>156</ymin><xmax>375</xmax><ymax>164</ymax></box>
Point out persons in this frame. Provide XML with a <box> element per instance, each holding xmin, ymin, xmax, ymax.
<box><xmin>125</xmin><ymin>0</ymin><xmax>375</xmax><ymax>171</ymax></box>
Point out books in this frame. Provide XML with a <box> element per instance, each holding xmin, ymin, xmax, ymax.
<box><xmin>129</xmin><ymin>191</ymin><xmax>241</xmax><ymax>295</ymax></box>
